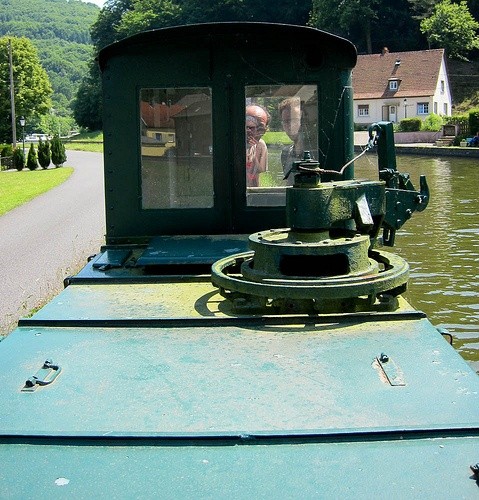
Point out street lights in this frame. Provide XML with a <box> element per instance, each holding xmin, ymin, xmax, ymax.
<box><xmin>20</xmin><ymin>115</ymin><xmax>26</xmax><ymax>155</ymax></box>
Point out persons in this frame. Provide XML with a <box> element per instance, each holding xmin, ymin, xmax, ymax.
<box><xmin>277</xmin><ymin>97</ymin><xmax>305</xmax><ymax>180</ymax></box>
<box><xmin>246</xmin><ymin>104</ymin><xmax>271</xmax><ymax>187</ymax></box>
